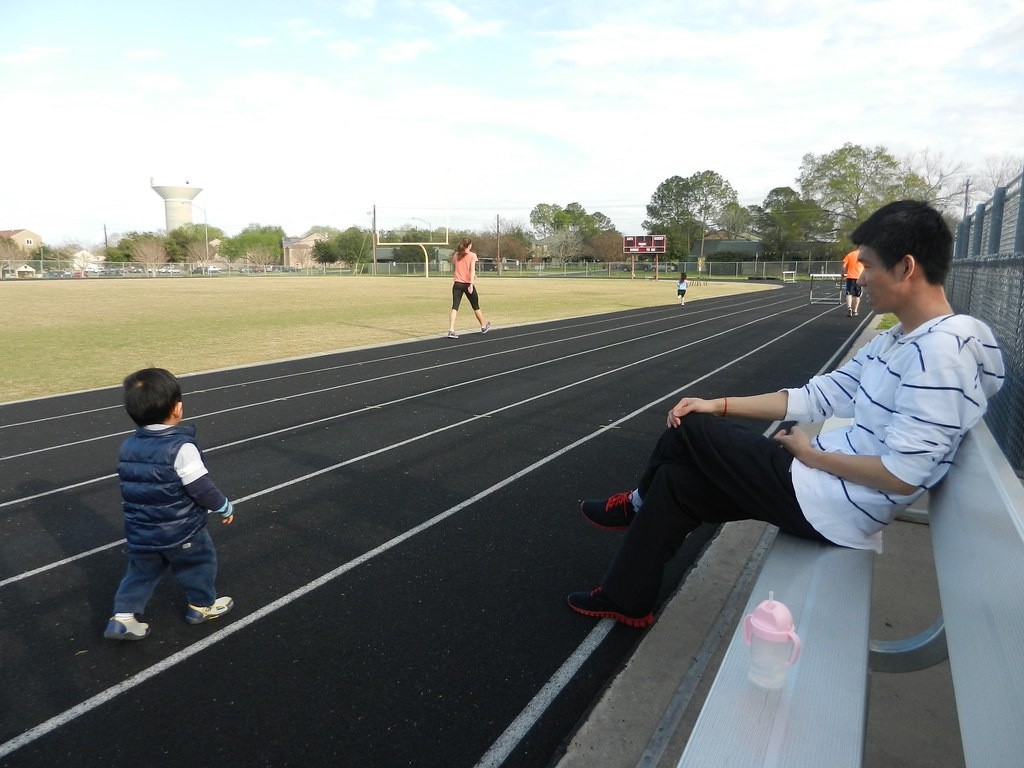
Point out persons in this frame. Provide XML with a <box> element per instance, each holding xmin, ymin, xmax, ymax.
<box><xmin>102</xmin><ymin>367</ymin><xmax>237</xmax><ymax>644</ymax></box>
<box><xmin>678</xmin><ymin>272</ymin><xmax>689</xmax><ymax>305</ymax></box>
<box><xmin>567</xmin><ymin>199</ymin><xmax>1000</xmax><ymax>628</ymax></box>
<box><xmin>446</xmin><ymin>237</ymin><xmax>492</xmax><ymax>339</ymax></box>
<box><xmin>839</xmin><ymin>245</ymin><xmax>864</xmax><ymax>318</ymax></box>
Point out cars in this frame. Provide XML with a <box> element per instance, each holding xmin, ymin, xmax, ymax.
<box><xmin>43</xmin><ymin>266</ymin><xmax>300</xmax><ymax>278</ymax></box>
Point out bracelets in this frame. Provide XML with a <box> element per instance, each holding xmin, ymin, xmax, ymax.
<box><xmin>722</xmin><ymin>397</ymin><xmax>728</xmax><ymax>417</ymax></box>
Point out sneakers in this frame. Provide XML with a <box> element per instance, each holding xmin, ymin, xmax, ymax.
<box><xmin>853</xmin><ymin>311</ymin><xmax>858</xmax><ymax>315</ymax></box>
<box><xmin>580</xmin><ymin>490</ymin><xmax>637</xmax><ymax>531</ymax></box>
<box><xmin>447</xmin><ymin>331</ymin><xmax>458</xmax><ymax>338</ymax></box>
<box><xmin>103</xmin><ymin>618</ymin><xmax>151</xmax><ymax>641</ymax></box>
<box><xmin>567</xmin><ymin>585</ymin><xmax>655</xmax><ymax>626</ymax></box>
<box><xmin>481</xmin><ymin>322</ymin><xmax>491</xmax><ymax>334</ymax></box>
<box><xmin>847</xmin><ymin>308</ymin><xmax>852</xmax><ymax>317</ymax></box>
<box><xmin>184</xmin><ymin>595</ymin><xmax>234</xmax><ymax>624</ymax></box>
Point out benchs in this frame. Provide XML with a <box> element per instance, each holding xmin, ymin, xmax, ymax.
<box><xmin>675</xmin><ymin>416</ymin><xmax>1024</xmax><ymax>767</ymax></box>
